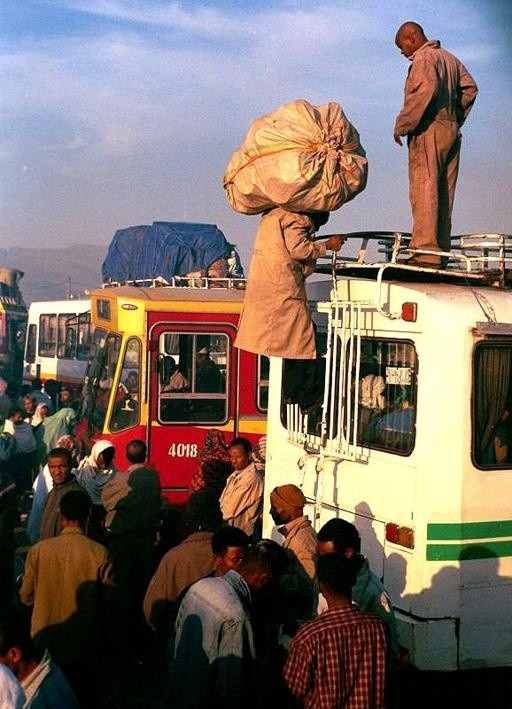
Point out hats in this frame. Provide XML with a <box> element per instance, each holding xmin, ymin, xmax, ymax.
<box><xmin>271</xmin><ymin>484</ymin><xmax>306</xmax><ymax>516</ymax></box>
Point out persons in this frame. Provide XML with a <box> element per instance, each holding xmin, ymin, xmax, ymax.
<box><xmin>393</xmin><ymin>21</ymin><xmax>479</xmax><ymax>267</ymax></box>
<box><xmin>278</xmin><ymin>553</ymin><xmax>394</xmax><ymax>709</ymax></box>
<box><xmin>19</xmin><ymin>490</ymin><xmax>116</xmax><ymax>708</ymax></box>
<box><xmin>268</xmin><ymin>484</ymin><xmax>317</xmax><ymax>578</ymax></box>
<box><xmin>219</xmin><ymin>438</ymin><xmax>265</xmax><ymax>536</ymax></box>
<box><xmin>495</xmin><ymin>402</ymin><xmax>510</xmax><ymax>463</ymax></box>
<box><xmin>165</xmin><ymin>538</ymin><xmax>281</xmax><ymax>708</ymax></box>
<box><xmin>124</xmin><ymin>504</ymin><xmax>188</xmax><ymax>707</ymax></box>
<box><xmin>2</xmin><ymin>369</ymin><xmax>148</xmax><ymax>580</ymax></box>
<box><xmin>231</xmin><ymin>208</ymin><xmax>347</xmax><ymax>414</ymax></box>
<box><xmin>1</xmin><ymin>603</ymin><xmax>80</xmax><ymax>708</ymax></box>
<box><xmin>309</xmin><ymin>371</ymin><xmax>414</xmax><ymax>449</ymax></box>
<box><xmin>110</xmin><ymin>464</ymin><xmax>182</xmax><ymax>584</ymax></box>
<box><xmin>174</xmin><ymin>525</ymin><xmax>248</xmax><ymax>619</ymax></box>
<box><xmin>310</xmin><ymin>518</ymin><xmax>400</xmax><ymax>677</ymax></box>
<box><xmin>160</xmin><ymin>345</ymin><xmax>225</xmax><ymax>422</ymax></box>
<box><xmin>142</xmin><ymin>492</ymin><xmax>224</xmax><ymax>626</ymax></box>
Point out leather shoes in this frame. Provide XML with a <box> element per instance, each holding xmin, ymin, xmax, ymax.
<box><xmin>398</xmin><ymin>258</ymin><xmax>441</xmax><ymax>268</ymax></box>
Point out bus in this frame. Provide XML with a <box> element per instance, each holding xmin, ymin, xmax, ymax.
<box><xmin>20</xmin><ymin>295</ymin><xmax>92</xmax><ymax>399</ymax></box>
<box><xmin>259</xmin><ymin>229</ymin><xmax>512</xmax><ymax>678</ymax></box>
<box><xmin>87</xmin><ymin>273</ymin><xmax>274</xmax><ymax>536</ymax></box>
